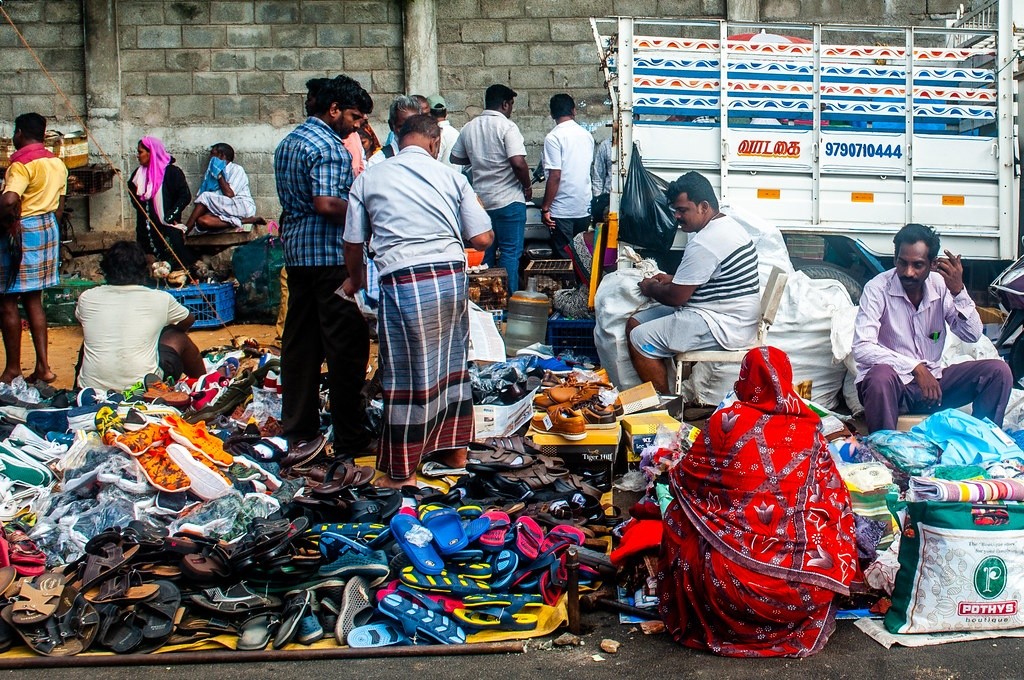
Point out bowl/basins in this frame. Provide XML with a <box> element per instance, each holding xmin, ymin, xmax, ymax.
<box><xmin>465</xmin><ymin>248</ymin><xmax>485</xmax><ymax>268</ymax></box>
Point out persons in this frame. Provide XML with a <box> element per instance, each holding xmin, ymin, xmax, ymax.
<box><xmin>127</xmin><ymin>137</ymin><xmax>192</xmax><ymax>288</ymax></box>
<box><xmin>852</xmin><ymin>223</ymin><xmax>1014</xmax><ymax>434</ymax></box>
<box><xmin>538</xmin><ymin>94</ymin><xmax>596</xmax><ymax>289</ymax></box>
<box><xmin>625</xmin><ymin>170</ymin><xmax>761</xmax><ymax>396</ymax></box>
<box><xmin>183</xmin><ymin>142</ymin><xmax>268</xmax><ymax>238</ymax></box>
<box><xmin>272</xmin><ymin>75</ymin><xmax>461</xmax><ymax>350</ymax></box>
<box><xmin>590</xmin><ymin>116</ymin><xmax>622</xmax><ymax>232</ymax></box>
<box><xmin>653</xmin><ymin>347</ymin><xmax>858</xmax><ymax>660</ymax></box>
<box><xmin>72</xmin><ymin>238</ymin><xmax>207</xmax><ymax>393</ymax></box>
<box><xmin>0</xmin><ymin>112</ymin><xmax>68</xmax><ymax>386</ymax></box>
<box><xmin>344</xmin><ymin>95</ymin><xmax>495</xmax><ymax>490</ymax></box>
<box><xmin>275</xmin><ymin>75</ymin><xmax>375</xmax><ymax>464</ymax></box>
<box><xmin>449</xmin><ymin>84</ymin><xmax>533</xmax><ymax>323</ymax></box>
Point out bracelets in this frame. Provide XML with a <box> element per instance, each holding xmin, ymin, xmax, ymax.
<box><xmin>217</xmin><ymin>174</ymin><xmax>222</xmax><ymax>179</ymax></box>
<box><xmin>524</xmin><ymin>183</ymin><xmax>532</xmax><ymax>191</ymax></box>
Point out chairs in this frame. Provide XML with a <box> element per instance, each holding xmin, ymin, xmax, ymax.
<box><xmin>675</xmin><ymin>266</ymin><xmax>787</xmax><ymax>394</ymax></box>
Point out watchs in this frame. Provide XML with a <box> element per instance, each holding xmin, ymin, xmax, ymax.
<box><xmin>540</xmin><ymin>208</ymin><xmax>550</xmax><ymax>215</ymax></box>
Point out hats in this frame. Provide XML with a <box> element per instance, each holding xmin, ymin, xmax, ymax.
<box><xmin>426</xmin><ymin>94</ymin><xmax>446</xmax><ymax>110</ymax></box>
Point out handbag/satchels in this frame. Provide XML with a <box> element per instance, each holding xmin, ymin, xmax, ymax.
<box><xmin>882</xmin><ymin>497</ymin><xmax>1024</xmax><ymax>634</ymax></box>
<box><xmin>616</xmin><ymin>141</ymin><xmax>679</xmax><ymax>255</ymax></box>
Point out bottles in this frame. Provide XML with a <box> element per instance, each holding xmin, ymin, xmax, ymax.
<box><xmin>504</xmin><ymin>277</ymin><xmax>550</xmax><ymax>357</ymax></box>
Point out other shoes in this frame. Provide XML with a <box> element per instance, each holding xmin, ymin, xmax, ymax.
<box><xmin>468</xmin><ymin>342</ymin><xmax>625</xmax><ymax>441</ymax></box>
<box><xmin>0</xmin><ymin>337</ymin><xmax>383</xmax><ymax>545</ymax></box>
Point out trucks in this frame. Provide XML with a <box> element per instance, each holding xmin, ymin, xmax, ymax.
<box><xmin>589</xmin><ymin>0</ymin><xmax>1024</xmax><ymax>302</ymax></box>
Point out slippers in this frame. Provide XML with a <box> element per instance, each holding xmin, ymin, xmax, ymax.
<box><xmin>24</xmin><ymin>372</ymin><xmax>57</xmax><ymax>383</ymax></box>
<box><xmin>1</xmin><ymin>437</ymin><xmax>624</xmax><ymax>655</ymax></box>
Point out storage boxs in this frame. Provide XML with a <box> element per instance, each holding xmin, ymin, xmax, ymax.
<box><xmin>547</xmin><ymin>309</ymin><xmax>600</xmax><ymax>367</ymax></box>
<box><xmin>153</xmin><ymin>283</ymin><xmax>235</xmax><ymax>328</ymax></box>
<box><xmin>523</xmin><ymin>381</ymin><xmax>682</xmax><ymax>473</ymax></box>
